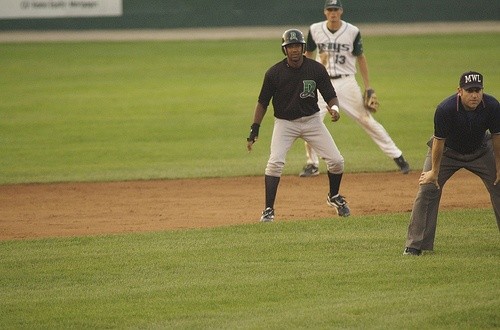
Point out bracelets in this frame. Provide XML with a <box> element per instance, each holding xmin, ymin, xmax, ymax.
<box><xmin>330</xmin><ymin>105</ymin><xmax>338</xmax><ymax>112</ymax></box>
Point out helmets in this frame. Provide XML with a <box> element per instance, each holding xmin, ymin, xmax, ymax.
<box><xmin>281</xmin><ymin>28</ymin><xmax>306</xmax><ymax>56</ymax></box>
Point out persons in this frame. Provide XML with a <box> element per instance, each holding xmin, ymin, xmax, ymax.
<box><xmin>402</xmin><ymin>70</ymin><xmax>500</xmax><ymax>255</ymax></box>
<box><xmin>246</xmin><ymin>29</ymin><xmax>351</xmax><ymax>221</ymax></box>
<box><xmin>298</xmin><ymin>0</ymin><xmax>409</xmax><ymax>177</ymax></box>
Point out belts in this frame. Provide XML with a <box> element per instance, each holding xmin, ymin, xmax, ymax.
<box><xmin>330</xmin><ymin>74</ymin><xmax>350</xmax><ymax>79</ymax></box>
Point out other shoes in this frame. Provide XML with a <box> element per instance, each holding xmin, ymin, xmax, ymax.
<box><xmin>402</xmin><ymin>164</ymin><xmax>410</xmax><ymax>174</ymax></box>
<box><xmin>326</xmin><ymin>192</ymin><xmax>351</xmax><ymax>217</ymax></box>
<box><xmin>259</xmin><ymin>207</ymin><xmax>274</xmax><ymax>222</ymax></box>
<box><xmin>403</xmin><ymin>247</ymin><xmax>423</xmax><ymax>258</ymax></box>
<box><xmin>299</xmin><ymin>164</ymin><xmax>320</xmax><ymax>177</ymax></box>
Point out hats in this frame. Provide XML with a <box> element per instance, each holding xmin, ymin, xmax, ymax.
<box><xmin>459</xmin><ymin>70</ymin><xmax>485</xmax><ymax>90</ymax></box>
<box><xmin>324</xmin><ymin>0</ymin><xmax>343</xmax><ymax>10</ymax></box>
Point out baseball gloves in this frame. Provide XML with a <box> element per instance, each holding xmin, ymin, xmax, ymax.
<box><xmin>364</xmin><ymin>89</ymin><xmax>378</xmax><ymax>112</ymax></box>
